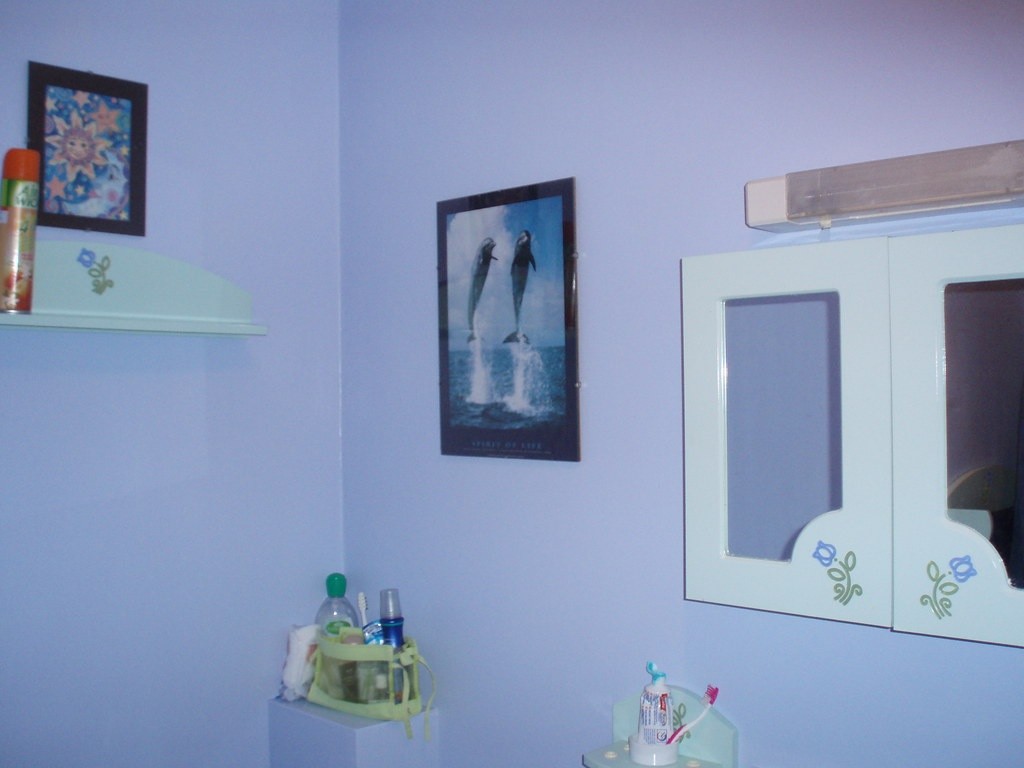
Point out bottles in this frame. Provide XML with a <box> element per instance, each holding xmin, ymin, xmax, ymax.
<box><xmin>0</xmin><ymin>148</ymin><xmax>41</xmax><ymax>315</ymax></box>
<box><xmin>315</xmin><ymin>573</ymin><xmax>358</xmax><ymax>638</ymax></box>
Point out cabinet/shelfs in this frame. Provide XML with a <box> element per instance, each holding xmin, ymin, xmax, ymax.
<box><xmin>680</xmin><ymin>224</ymin><xmax>1023</xmax><ymax>646</ymax></box>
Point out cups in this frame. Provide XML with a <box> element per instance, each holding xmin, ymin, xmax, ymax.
<box><xmin>629</xmin><ymin>733</ymin><xmax>678</xmax><ymax>766</ymax></box>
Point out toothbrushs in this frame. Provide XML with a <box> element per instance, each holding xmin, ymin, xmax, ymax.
<box><xmin>667</xmin><ymin>682</ymin><xmax>720</xmax><ymax>744</ymax></box>
<box><xmin>355</xmin><ymin>590</ymin><xmax>369</xmax><ymax>625</ymax></box>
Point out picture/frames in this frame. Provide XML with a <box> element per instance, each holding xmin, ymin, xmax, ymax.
<box><xmin>437</xmin><ymin>177</ymin><xmax>581</xmax><ymax>463</ymax></box>
<box><xmin>26</xmin><ymin>59</ymin><xmax>149</xmax><ymax>237</ymax></box>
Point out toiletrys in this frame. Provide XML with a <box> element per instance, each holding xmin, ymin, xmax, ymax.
<box><xmin>315</xmin><ymin>572</ymin><xmax>409</xmax><ymax>707</ymax></box>
<box><xmin>637</xmin><ymin>659</ymin><xmax>675</xmax><ymax>745</ymax></box>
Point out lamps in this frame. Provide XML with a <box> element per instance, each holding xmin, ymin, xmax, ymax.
<box><xmin>744</xmin><ymin>140</ymin><xmax>1024</xmax><ymax>232</ymax></box>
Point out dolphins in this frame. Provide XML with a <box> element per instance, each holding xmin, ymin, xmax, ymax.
<box><xmin>464</xmin><ymin>229</ymin><xmax>538</xmax><ymax>346</ymax></box>
